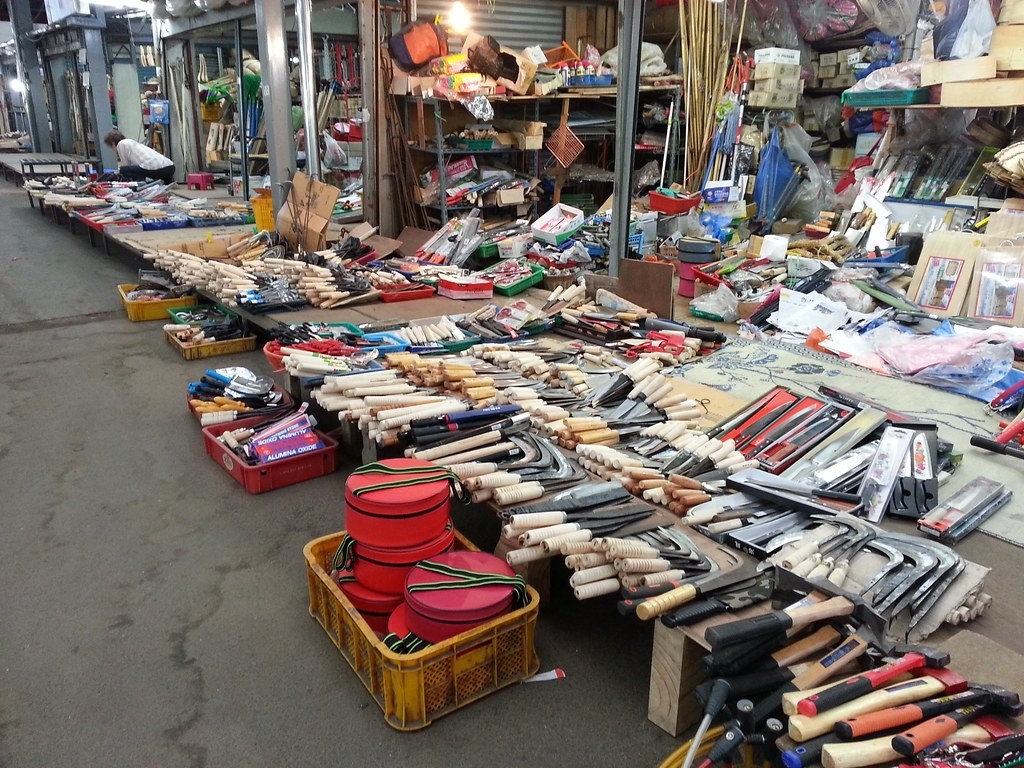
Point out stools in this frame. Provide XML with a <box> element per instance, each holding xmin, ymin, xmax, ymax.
<box><xmin>185</xmin><ymin>172</ymin><xmax>215</xmax><ymax>191</ymax></box>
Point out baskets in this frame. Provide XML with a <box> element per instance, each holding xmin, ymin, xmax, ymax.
<box><xmin>166</xmin><ymin>302</ymin><xmax>239</xmax><ymax>325</ymax></box>
<box><xmin>186</xmin><ymin>376</ymin><xmax>295</xmax><ymax>430</ymax></box>
<box><xmin>201</xmin><ymin>411</ymin><xmax>339</xmax><ymax>494</ymax></box>
<box><xmin>164</xmin><ymin>317</ymin><xmax>258</xmax><ymax>359</ymax></box>
<box><xmin>303</xmin><ymin>518</ymin><xmax>542</xmax><ymax>732</ymax></box>
<box><xmin>117</xmin><ymin>283</ymin><xmax>199</xmax><ymax>321</ymax></box>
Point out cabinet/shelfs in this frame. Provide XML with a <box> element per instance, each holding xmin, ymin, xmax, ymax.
<box><xmin>18</xmin><ymin>154</ymin><xmax>106</xmax><ymax>216</ymax></box>
<box><xmin>403</xmin><ymin>90</ymin><xmax>539</xmax><ymax>231</ymax></box>
<box><xmin>529</xmin><ymin>84</ymin><xmax>690</xmax><ymax>217</ymax></box>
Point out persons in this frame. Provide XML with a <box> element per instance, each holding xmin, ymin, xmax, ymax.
<box><xmin>103</xmin><ymin>130</ymin><xmax>176</xmax><ymax>185</ymax></box>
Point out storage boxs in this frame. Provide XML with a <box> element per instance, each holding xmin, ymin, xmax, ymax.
<box><xmin>167</xmin><ymin>306</ymin><xmax>240</xmax><ymax>324</ymax></box>
<box><xmin>200</xmin><ymin>102</ymin><xmax>224</xmax><ymax>122</ymax></box>
<box><xmin>262</xmin><ymin>310</ymin><xmax>532</xmax><ymax>374</ymax></box>
<box><xmin>373</xmin><ymin>221</ymin><xmax>645</xmax><ymax>304</ymax></box>
<box><xmin>162</xmin><ymin>324</ymin><xmax>257</xmax><ymax>361</ymax></box>
<box><xmin>117</xmin><ymin>283</ymin><xmax>198</xmax><ymax>322</ymax></box>
<box><xmin>842</xmin><ymin>89</ymin><xmax>932</xmax><ymax>107</ymax></box>
<box><xmin>202</xmin><ymin>411</ymin><xmax>341</xmax><ymax>497</ymax></box>
<box><xmin>302</xmin><ymin>521</ymin><xmax>541</xmax><ymax>733</ymax></box>
<box><xmin>646</xmin><ymin>191</ymin><xmax>704</xmax><ymax>217</ymax></box>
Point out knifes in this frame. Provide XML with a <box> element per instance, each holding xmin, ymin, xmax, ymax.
<box><xmin>129</xmin><ymin>228</ymin><xmax>953</xmax><ymax>635</ymax></box>
